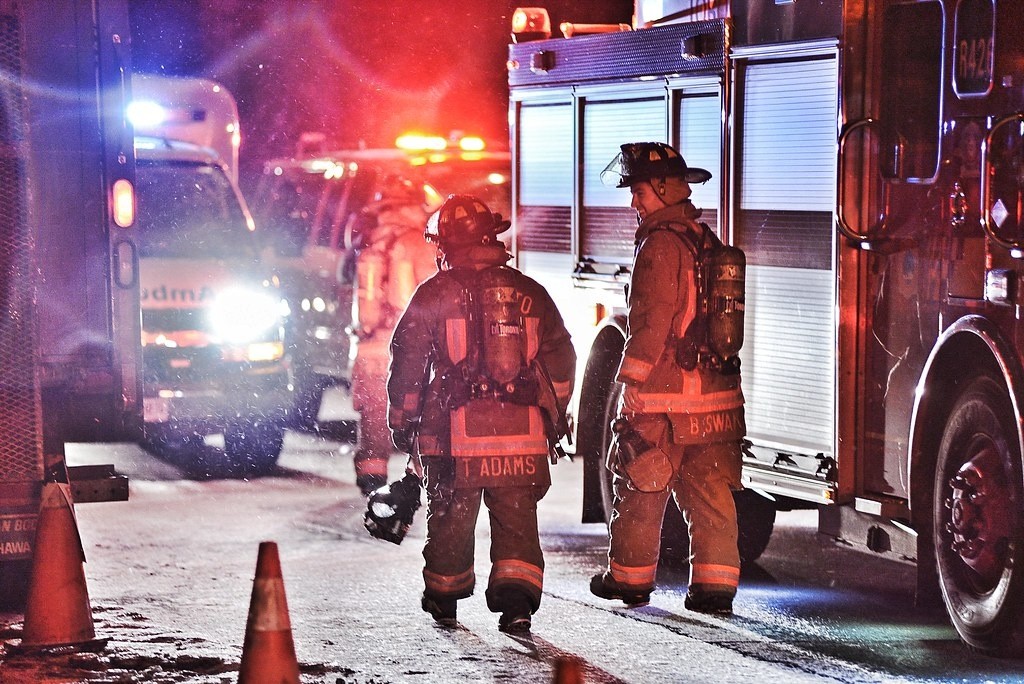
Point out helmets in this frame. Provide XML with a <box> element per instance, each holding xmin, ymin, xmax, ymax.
<box><xmin>366</xmin><ymin>173</ymin><xmax>424</xmax><ymax>215</ymax></box>
<box><xmin>424</xmin><ymin>194</ymin><xmax>512</xmax><ymax>245</ymax></box>
<box><xmin>600</xmin><ymin>143</ymin><xmax>712</xmax><ymax>190</ymax></box>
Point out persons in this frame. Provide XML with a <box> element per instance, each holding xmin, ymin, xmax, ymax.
<box><xmin>352</xmin><ymin>188</ymin><xmax>444</xmax><ymax>501</ymax></box>
<box><xmin>590</xmin><ymin>142</ymin><xmax>744</xmax><ymax>616</ymax></box>
<box><xmin>387</xmin><ymin>195</ymin><xmax>577</xmax><ymax>633</ymax></box>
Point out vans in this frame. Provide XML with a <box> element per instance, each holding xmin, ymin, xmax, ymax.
<box><xmin>249</xmin><ymin>140</ymin><xmax>511</xmax><ymax>432</ymax></box>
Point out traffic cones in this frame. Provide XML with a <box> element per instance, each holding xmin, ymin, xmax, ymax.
<box><xmin>237</xmin><ymin>541</ymin><xmax>301</xmax><ymax>684</ymax></box>
<box><xmin>3</xmin><ymin>456</ymin><xmax>113</xmax><ymax>653</ymax></box>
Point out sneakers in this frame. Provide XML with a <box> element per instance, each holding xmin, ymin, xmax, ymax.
<box><xmin>684</xmin><ymin>591</ymin><xmax>733</xmax><ymax>613</ymax></box>
<box><xmin>590</xmin><ymin>573</ymin><xmax>650</xmax><ymax>606</ymax></box>
<box><xmin>420</xmin><ymin>595</ymin><xmax>457</xmax><ymax>625</ymax></box>
<box><xmin>498</xmin><ymin>610</ymin><xmax>532</xmax><ymax>632</ymax></box>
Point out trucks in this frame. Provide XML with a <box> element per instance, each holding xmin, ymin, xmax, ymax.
<box><xmin>128</xmin><ymin>75</ymin><xmax>292</xmax><ymax>472</ymax></box>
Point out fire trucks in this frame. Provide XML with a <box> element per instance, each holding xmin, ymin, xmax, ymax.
<box><xmin>507</xmin><ymin>0</ymin><xmax>1023</xmax><ymax>656</ymax></box>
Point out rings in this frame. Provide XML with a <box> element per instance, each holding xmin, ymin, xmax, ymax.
<box><xmin>631</xmin><ymin>400</ymin><xmax>634</xmax><ymax>403</ymax></box>
